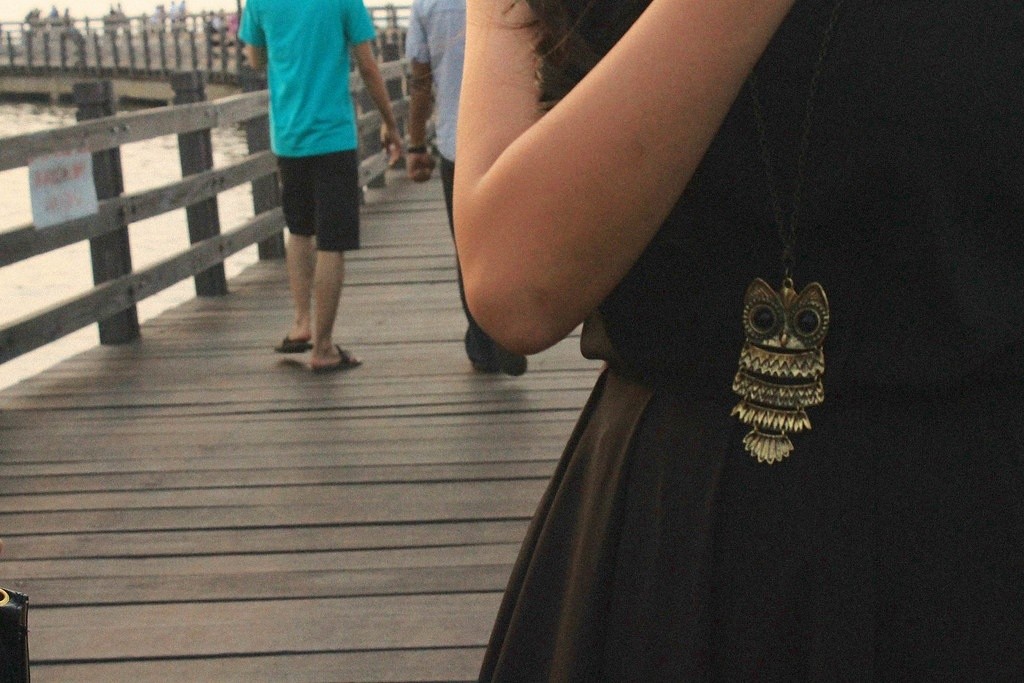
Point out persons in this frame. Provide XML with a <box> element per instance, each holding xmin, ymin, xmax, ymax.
<box><xmin>154</xmin><ymin>0</ymin><xmax>189</xmax><ymax>33</ymax></box>
<box><xmin>25</xmin><ymin>5</ymin><xmax>70</xmax><ymax>39</ymax></box>
<box><xmin>402</xmin><ymin>0</ymin><xmax>529</xmax><ymax>375</ymax></box>
<box><xmin>110</xmin><ymin>3</ymin><xmax>125</xmax><ymax>30</ymax></box>
<box><xmin>237</xmin><ymin>0</ymin><xmax>406</xmax><ymax>368</ymax></box>
<box><xmin>207</xmin><ymin>9</ymin><xmax>237</xmax><ymax>44</ymax></box>
<box><xmin>452</xmin><ymin>1</ymin><xmax>1024</xmax><ymax>683</ymax></box>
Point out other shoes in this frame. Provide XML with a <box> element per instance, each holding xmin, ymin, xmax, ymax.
<box><xmin>463</xmin><ymin>327</ymin><xmax>527</xmax><ymax>378</ymax></box>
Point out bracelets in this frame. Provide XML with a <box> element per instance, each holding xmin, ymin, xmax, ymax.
<box><xmin>406</xmin><ymin>145</ymin><xmax>426</xmax><ymax>153</ymax></box>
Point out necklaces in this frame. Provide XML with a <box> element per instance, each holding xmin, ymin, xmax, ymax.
<box><xmin>725</xmin><ymin>1</ymin><xmax>841</xmax><ymax>465</ymax></box>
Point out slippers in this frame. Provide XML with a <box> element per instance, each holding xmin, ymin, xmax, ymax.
<box><xmin>314</xmin><ymin>346</ymin><xmax>360</xmax><ymax>373</ymax></box>
<box><xmin>274</xmin><ymin>333</ymin><xmax>314</xmax><ymax>354</ymax></box>
